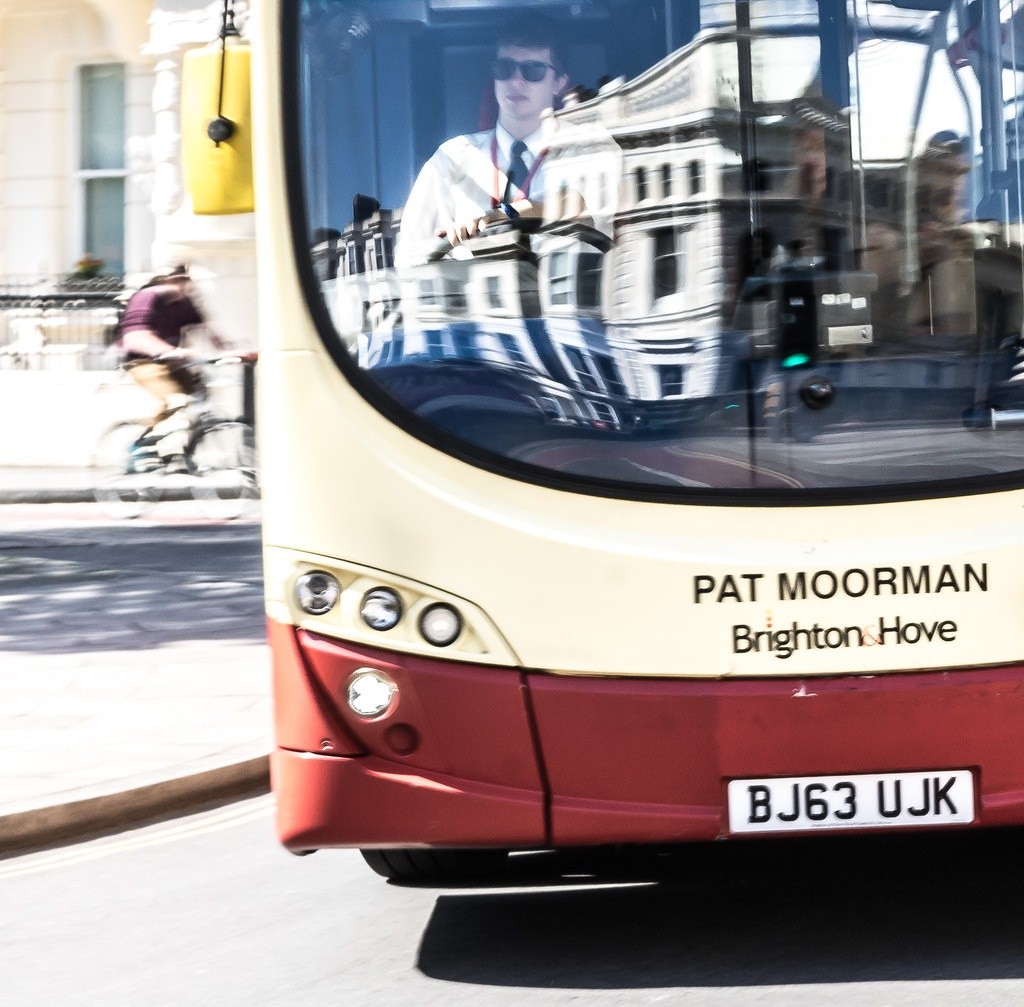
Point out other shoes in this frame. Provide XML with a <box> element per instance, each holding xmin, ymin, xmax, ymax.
<box><xmin>127</xmin><ymin>445</ymin><xmax>165</xmax><ymax>473</ymax></box>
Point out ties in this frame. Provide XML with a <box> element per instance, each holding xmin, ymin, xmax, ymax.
<box><xmin>504</xmin><ymin>141</ymin><xmax>530</xmax><ymax>203</ymax></box>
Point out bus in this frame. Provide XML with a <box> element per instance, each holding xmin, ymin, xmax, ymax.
<box><xmin>179</xmin><ymin>0</ymin><xmax>1023</xmax><ymax>899</ymax></box>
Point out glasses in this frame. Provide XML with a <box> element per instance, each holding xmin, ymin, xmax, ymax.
<box><xmin>487</xmin><ymin>57</ymin><xmax>560</xmax><ymax>82</ymax></box>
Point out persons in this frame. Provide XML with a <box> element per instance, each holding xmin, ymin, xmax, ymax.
<box><xmin>394</xmin><ymin>15</ymin><xmax>625</xmax><ymax>354</ymax></box>
<box><xmin>118</xmin><ymin>259</ymin><xmax>225</xmax><ymax>478</ymax></box>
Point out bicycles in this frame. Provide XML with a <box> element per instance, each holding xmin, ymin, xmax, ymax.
<box><xmin>84</xmin><ymin>347</ymin><xmax>256</xmax><ymax>477</ymax></box>
